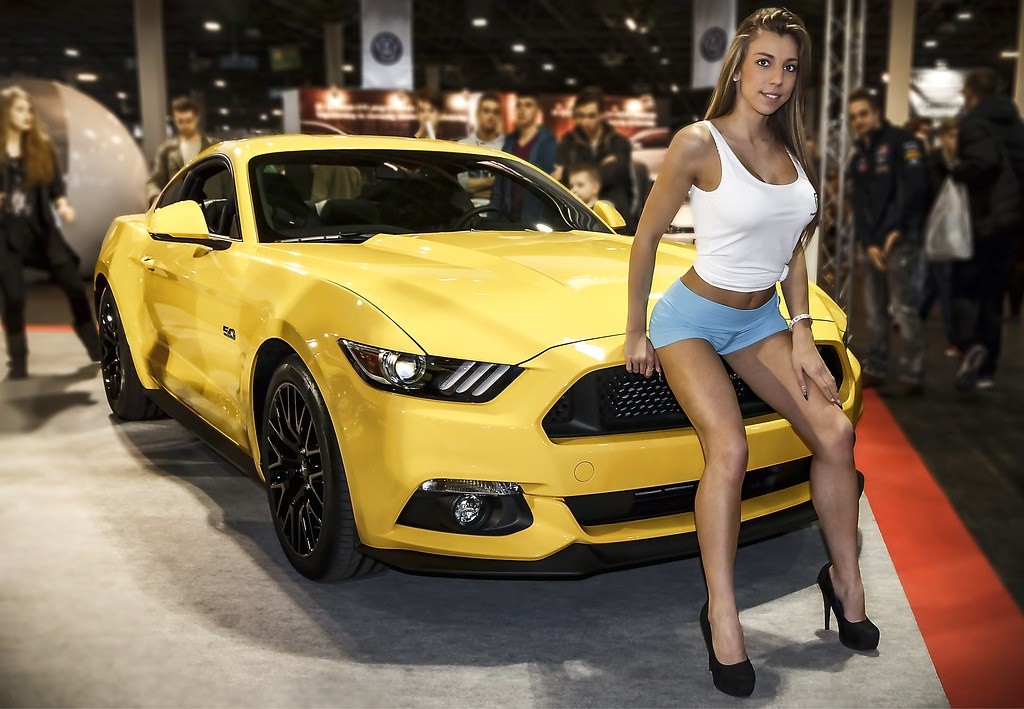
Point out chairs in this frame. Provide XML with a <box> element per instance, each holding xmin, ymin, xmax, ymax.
<box><xmin>217</xmin><ymin>173</ymin><xmax>318</xmax><ymax>239</ymax></box>
<box><xmin>363</xmin><ymin>164</ymin><xmax>481</xmax><ymax>231</ymax></box>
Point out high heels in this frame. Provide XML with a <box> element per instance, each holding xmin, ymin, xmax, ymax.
<box><xmin>700</xmin><ymin>601</ymin><xmax>757</xmax><ymax>697</ymax></box>
<box><xmin>817</xmin><ymin>561</ymin><xmax>881</xmax><ymax>649</ymax></box>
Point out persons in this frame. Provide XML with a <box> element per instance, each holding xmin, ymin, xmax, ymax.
<box><xmin>145</xmin><ymin>97</ymin><xmax>233</xmax><ymax>228</ymax></box>
<box><xmin>842</xmin><ymin>88</ymin><xmax>929</xmax><ymax>386</ymax></box>
<box><xmin>303</xmin><ymin>162</ymin><xmax>362</xmax><ymax>207</ymax></box>
<box><xmin>0</xmin><ymin>86</ymin><xmax>102</xmax><ymax>380</ymax></box>
<box><xmin>569</xmin><ymin>164</ymin><xmax>614</xmax><ymax>210</ymax></box>
<box><xmin>410</xmin><ymin>90</ymin><xmax>447</xmax><ymax>144</ymax></box>
<box><xmin>948</xmin><ymin>67</ymin><xmax>1023</xmax><ymax>388</ymax></box>
<box><xmin>550</xmin><ymin>91</ymin><xmax>632</xmax><ymax>215</ymax></box>
<box><xmin>485</xmin><ymin>92</ymin><xmax>560</xmax><ymax>229</ymax></box>
<box><xmin>626</xmin><ymin>8</ymin><xmax>879</xmax><ymax>699</ymax></box>
<box><xmin>450</xmin><ymin>93</ymin><xmax>504</xmax><ymax>219</ymax></box>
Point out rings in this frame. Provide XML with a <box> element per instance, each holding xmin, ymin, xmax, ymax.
<box><xmin>646</xmin><ymin>367</ymin><xmax>654</xmax><ymax>372</ymax></box>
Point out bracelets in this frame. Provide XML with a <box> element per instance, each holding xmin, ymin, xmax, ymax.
<box><xmin>788</xmin><ymin>314</ymin><xmax>814</xmax><ymax>332</ymax></box>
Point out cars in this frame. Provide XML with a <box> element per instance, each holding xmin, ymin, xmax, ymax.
<box><xmin>94</xmin><ymin>132</ymin><xmax>868</xmax><ymax>584</ymax></box>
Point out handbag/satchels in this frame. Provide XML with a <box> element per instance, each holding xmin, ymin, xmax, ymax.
<box><xmin>922</xmin><ymin>174</ymin><xmax>974</xmax><ymax>260</ymax></box>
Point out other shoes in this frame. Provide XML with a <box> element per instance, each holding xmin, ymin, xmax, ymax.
<box><xmin>957</xmin><ymin>346</ymin><xmax>993</xmax><ymax>391</ymax></box>
<box><xmin>860</xmin><ymin>372</ymin><xmax>925</xmax><ymax>399</ymax></box>
<box><xmin>74</xmin><ymin>320</ymin><xmax>106</xmax><ymax>361</ymax></box>
<box><xmin>8</xmin><ymin>356</ymin><xmax>26</xmax><ymax>376</ymax></box>
